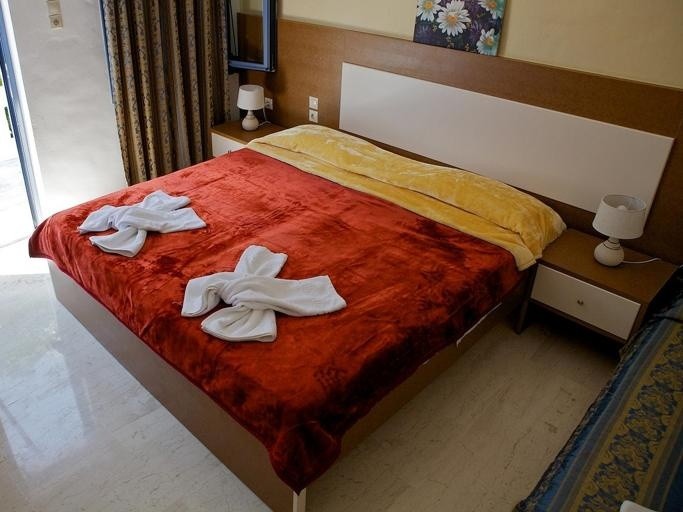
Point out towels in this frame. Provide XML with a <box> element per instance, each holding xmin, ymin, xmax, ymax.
<box><xmin>75</xmin><ymin>188</ymin><xmax>209</xmax><ymax>259</ymax></box>
<box><xmin>180</xmin><ymin>244</ymin><xmax>348</xmax><ymax>345</ymax></box>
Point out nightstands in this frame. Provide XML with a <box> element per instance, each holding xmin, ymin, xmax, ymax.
<box><xmin>528</xmin><ymin>226</ymin><xmax>676</xmax><ymax>357</ymax></box>
<box><xmin>208</xmin><ymin>121</ymin><xmax>285</xmax><ymax>158</ymax></box>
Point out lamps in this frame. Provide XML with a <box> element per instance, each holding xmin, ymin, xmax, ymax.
<box><xmin>235</xmin><ymin>81</ymin><xmax>269</xmax><ymax>132</ymax></box>
<box><xmin>589</xmin><ymin>188</ymin><xmax>648</xmax><ymax>267</ymax></box>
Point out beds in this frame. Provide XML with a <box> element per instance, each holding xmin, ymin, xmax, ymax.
<box><xmin>26</xmin><ymin>122</ymin><xmax>567</xmax><ymax>512</ymax></box>
<box><xmin>506</xmin><ymin>284</ymin><xmax>683</xmax><ymax>512</ymax></box>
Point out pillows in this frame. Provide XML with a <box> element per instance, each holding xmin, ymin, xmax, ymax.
<box><xmin>246</xmin><ymin>122</ymin><xmax>566</xmax><ymax>272</ymax></box>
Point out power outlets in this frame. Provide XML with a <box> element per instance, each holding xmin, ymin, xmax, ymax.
<box><xmin>263</xmin><ymin>97</ymin><xmax>274</xmax><ymax>112</ymax></box>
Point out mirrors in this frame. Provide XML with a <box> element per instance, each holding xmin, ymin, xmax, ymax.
<box><xmin>227</xmin><ymin>0</ymin><xmax>277</xmax><ymax>73</ymax></box>
